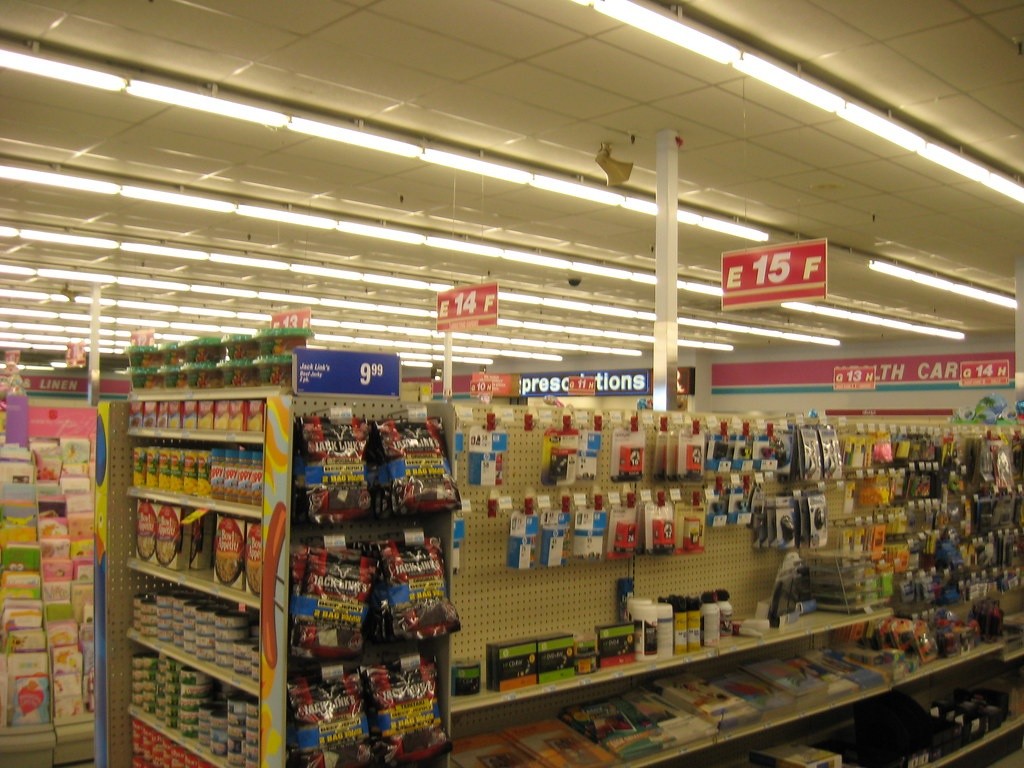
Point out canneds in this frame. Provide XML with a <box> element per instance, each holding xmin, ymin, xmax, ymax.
<box><xmin>132</xmin><ymin>445</ymin><xmax>264</xmax><ymax>508</ymax></box>
<box><xmin>130</xmin><ymin>590</ymin><xmax>261</xmax><ymax>768</ymax></box>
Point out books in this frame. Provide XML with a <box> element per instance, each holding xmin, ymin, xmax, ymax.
<box><xmin>450</xmin><ymin>717</ymin><xmax>619</xmax><ymax>768</ymax></box>
<box><xmin>0</xmin><ymin>435</ymin><xmax>96</xmax><ymax>726</ymax></box>
<box><xmin>786</xmin><ymin>647</ymin><xmax>860</xmax><ymax>705</ymax></box>
<box><xmin>557</xmin><ymin>684</ymin><xmax>719</xmax><ymax>762</ymax></box>
<box><xmin>648</xmin><ymin>654</ymin><xmax>830</xmax><ymax>730</ymax></box>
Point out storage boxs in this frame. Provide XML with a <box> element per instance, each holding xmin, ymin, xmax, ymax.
<box><xmin>530</xmin><ymin>631</ymin><xmax>575</xmax><ymax>684</ymax></box>
<box><xmin>594</xmin><ymin>622</ymin><xmax>636</xmax><ymax>669</ymax></box>
<box><xmin>486</xmin><ymin>637</ymin><xmax>538</xmax><ymax>693</ymax></box>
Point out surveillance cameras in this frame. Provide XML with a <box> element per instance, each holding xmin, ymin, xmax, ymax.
<box><xmin>569</xmin><ymin>279</ymin><xmax>580</xmax><ymax>286</ymax></box>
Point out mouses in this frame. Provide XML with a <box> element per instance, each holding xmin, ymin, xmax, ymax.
<box><xmin>806</xmin><ymin>454</ymin><xmax>818</xmax><ymax>478</ymax></box>
<box><xmin>781</xmin><ymin>516</ymin><xmax>794</xmax><ymax>542</ymax></box>
<box><xmin>814</xmin><ymin>508</ymin><xmax>824</xmax><ymax>529</ymax></box>
<box><xmin>828</xmin><ymin>457</ymin><xmax>837</xmax><ymax>477</ymax></box>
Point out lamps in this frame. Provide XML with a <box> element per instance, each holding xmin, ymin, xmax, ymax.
<box><xmin>0</xmin><ymin>0</ymin><xmax>1024</xmax><ymax>371</ymax></box>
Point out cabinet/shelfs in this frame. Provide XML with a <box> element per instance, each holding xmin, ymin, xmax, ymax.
<box><xmin>93</xmin><ymin>329</ymin><xmax>1024</xmax><ymax>768</ymax></box>
<box><xmin>0</xmin><ymin>374</ymin><xmax>93</xmax><ymax>768</ymax></box>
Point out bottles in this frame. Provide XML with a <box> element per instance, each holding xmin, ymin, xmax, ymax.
<box><xmin>667</xmin><ymin>594</ymin><xmax>701</xmax><ymax>655</ymax></box>
<box><xmin>700</xmin><ymin>590</ymin><xmax>720</xmax><ymax>647</ymax></box>
<box><xmin>628</xmin><ymin>596</ymin><xmax>673</xmax><ymax>661</ymax></box>
<box><xmin>716</xmin><ymin>589</ymin><xmax>734</xmax><ymax>637</ymax></box>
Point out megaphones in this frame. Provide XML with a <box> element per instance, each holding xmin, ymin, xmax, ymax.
<box><xmin>594</xmin><ymin>150</ymin><xmax>633</xmax><ymax>186</ymax></box>
